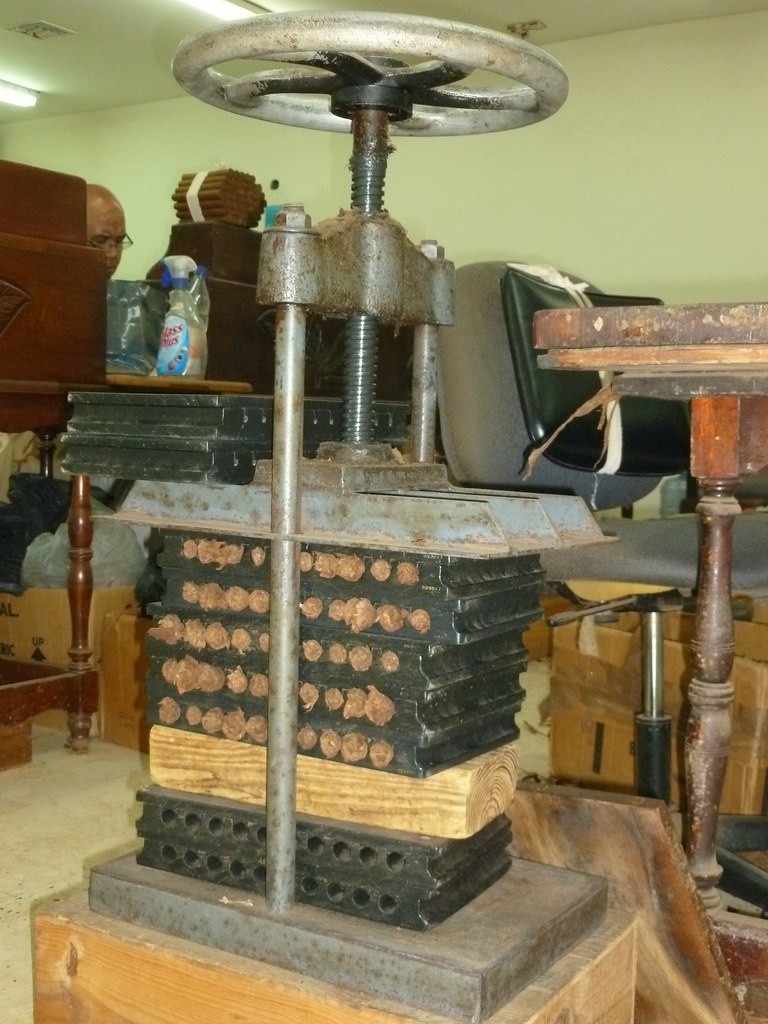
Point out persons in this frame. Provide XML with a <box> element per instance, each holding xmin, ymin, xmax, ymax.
<box><xmin>83</xmin><ymin>182</ymin><xmax>127</xmax><ymax>280</ymax></box>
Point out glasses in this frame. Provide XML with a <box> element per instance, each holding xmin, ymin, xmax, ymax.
<box><xmin>86</xmin><ymin>234</ymin><xmax>134</xmax><ymax>251</ymax></box>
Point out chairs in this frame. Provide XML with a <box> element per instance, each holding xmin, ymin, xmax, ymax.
<box><xmin>426</xmin><ymin>260</ymin><xmax>768</xmax><ymax>913</ymax></box>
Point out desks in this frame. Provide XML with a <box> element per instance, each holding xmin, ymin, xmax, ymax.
<box><xmin>0</xmin><ymin>380</ymin><xmax>113</xmax><ymax>754</ymax></box>
<box><xmin>532</xmin><ymin>301</ymin><xmax>768</xmax><ymax>922</ymax></box>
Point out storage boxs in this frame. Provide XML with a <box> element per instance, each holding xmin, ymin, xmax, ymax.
<box><xmin>99</xmin><ymin>611</ymin><xmax>159</xmax><ymax>755</ymax></box>
<box><xmin>522</xmin><ymin>577</ymin><xmax>768</xmax><ymax>816</ymax></box>
<box><xmin>548</xmin><ymin>610</ymin><xmax>768</xmax><ymax>817</ymax></box>
<box><xmin>0</xmin><ymin>586</ymin><xmax>141</xmax><ymax>737</ymax></box>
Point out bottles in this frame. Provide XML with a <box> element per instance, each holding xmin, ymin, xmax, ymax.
<box><xmin>191</xmin><ymin>267</ymin><xmax>211</xmax><ymax>328</ymax></box>
